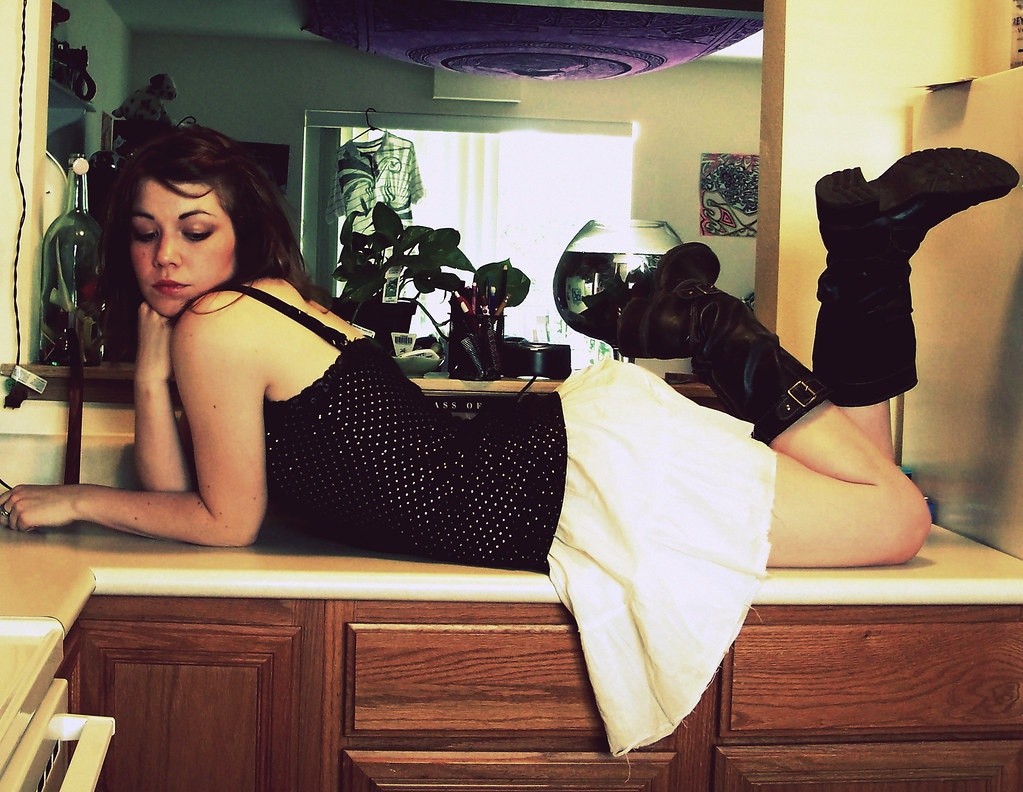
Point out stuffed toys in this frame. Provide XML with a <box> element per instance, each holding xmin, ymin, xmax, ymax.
<box><xmin>112</xmin><ymin>73</ymin><xmax>177</xmax><ymax>124</ymax></box>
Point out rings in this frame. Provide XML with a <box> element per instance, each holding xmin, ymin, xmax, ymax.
<box><xmin>1</xmin><ymin>508</ymin><xmax>10</xmax><ymax>517</ymax></box>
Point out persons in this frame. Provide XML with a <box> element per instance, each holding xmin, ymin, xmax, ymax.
<box><xmin>0</xmin><ymin>127</ymin><xmax>1020</xmax><ymax>758</ymax></box>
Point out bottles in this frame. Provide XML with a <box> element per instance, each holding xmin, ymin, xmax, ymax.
<box><xmin>40</xmin><ymin>152</ymin><xmax>105</xmax><ymax>367</ymax></box>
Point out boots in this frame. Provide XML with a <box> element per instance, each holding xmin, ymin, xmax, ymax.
<box><xmin>811</xmin><ymin>144</ymin><xmax>1023</xmax><ymax>408</ymax></box>
<box><xmin>617</xmin><ymin>242</ymin><xmax>834</xmax><ymax>445</ymax></box>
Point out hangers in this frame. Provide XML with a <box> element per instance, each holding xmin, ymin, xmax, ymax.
<box><xmin>338</xmin><ymin>108</ymin><xmax>412</xmax><ymax>155</ymax></box>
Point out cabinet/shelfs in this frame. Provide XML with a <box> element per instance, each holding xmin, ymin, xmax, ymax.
<box><xmin>58</xmin><ymin>597</ymin><xmax>1023</xmax><ymax>791</ymax></box>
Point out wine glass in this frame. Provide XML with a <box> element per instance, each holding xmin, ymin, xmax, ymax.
<box><xmin>553</xmin><ymin>221</ymin><xmax>683</xmax><ymax>363</ymax></box>
